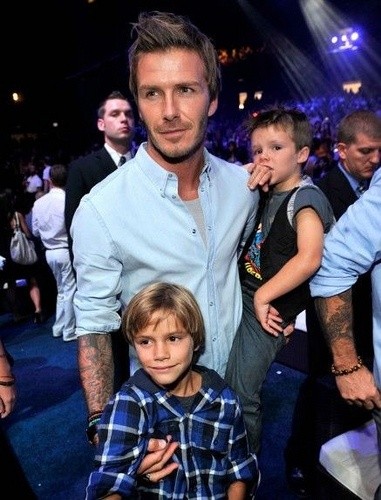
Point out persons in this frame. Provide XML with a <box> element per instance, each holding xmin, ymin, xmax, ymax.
<box><xmin>1</xmin><ymin>13</ymin><xmax>381</xmax><ymax>500</ymax></box>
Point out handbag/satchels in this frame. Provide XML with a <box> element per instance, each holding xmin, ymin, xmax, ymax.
<box><xmin>10</xmin><ymin>210</ymin><xmax>39</xmax><ymax>266</ymax></box>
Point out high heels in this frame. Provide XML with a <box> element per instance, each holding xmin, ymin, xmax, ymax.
<box><xmin>34</xmin><ymin>311</ymin><xmax>43</xmax><ymax>324</ymax></box>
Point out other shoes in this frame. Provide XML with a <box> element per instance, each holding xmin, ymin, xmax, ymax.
<box><xmin>284</xmin><ymin>465</ymin><xmax>310</xmax><ymax>497</ymax></box>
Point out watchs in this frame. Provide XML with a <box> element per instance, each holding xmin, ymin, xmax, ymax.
<box><xmin>85</xmin><ymin>425</ymin><xmax>98</xmax><ymax>444</ymax></box>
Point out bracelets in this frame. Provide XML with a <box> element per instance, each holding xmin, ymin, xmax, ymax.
<box><xmin>88</xmin><ymin>414</ymin><xmax>103</xmax><ymax>423</ymax></box>
<box><xmin>0</xmin><ymin>376</ymin><xmax>13</xmax><ymax>378</ymax></box>
<box><xmin>333</xmin><ymin>356</ymin><xmax>363</xmax><ymax>376</ymax></box>
<box><xmin>87</xmin><ymin>409</ymin><xmax>105</xmax><ymax>418</ymax></box>
<box><xmin>0</xmin><ymin>379</ymin><xmax>16</xmax><ymax>386</ymax></box>
